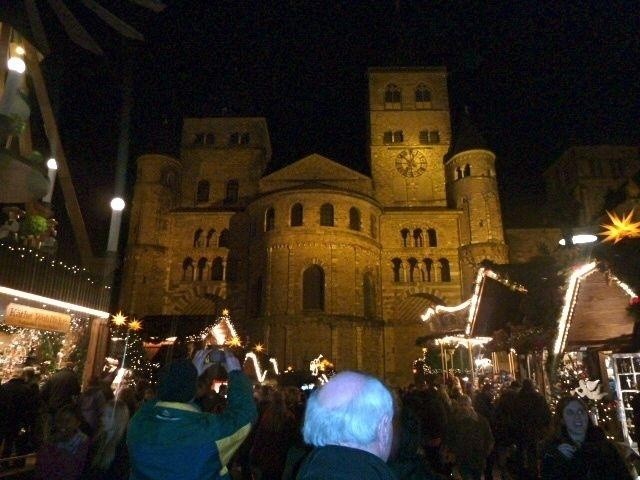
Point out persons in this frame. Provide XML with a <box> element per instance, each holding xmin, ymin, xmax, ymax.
<box><xmin>1</xmin><ymin>349</ymin><xmax>312</xmax><ymax>479</ymax></box>
<box><xmin>297</xmin><ymin>372</ymin><xmax>398</xmax><ymax>479</ymax></box>
<box><xmin>392</xmin><ymin>378</ymin><xmax>638</xmax><ymax>479</ymax></box>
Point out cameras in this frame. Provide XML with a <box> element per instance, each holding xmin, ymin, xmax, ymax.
<box><xmin>209</xmin><ymin>352</ymin><xmax>227</xmax><ymax>363</ymax></box>
